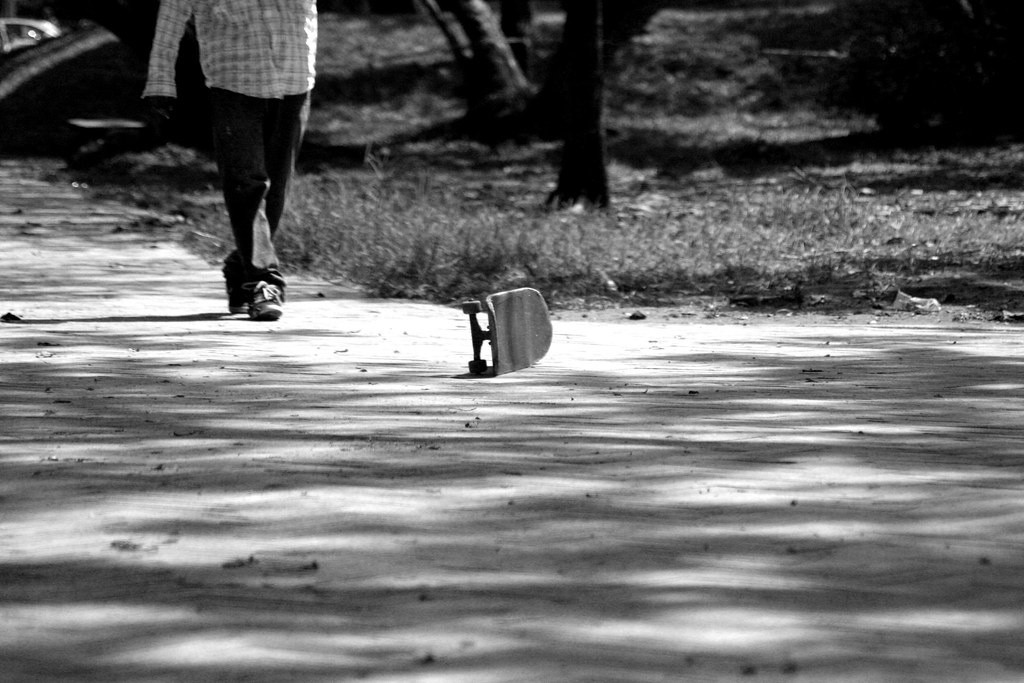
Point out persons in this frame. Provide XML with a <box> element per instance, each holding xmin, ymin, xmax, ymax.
<box><xmin>140</xmin><ymin>1</ymin><xmax>318</xmax><ymax>322</ymax></box>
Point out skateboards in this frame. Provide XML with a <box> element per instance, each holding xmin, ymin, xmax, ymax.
<box><xmin>461</xmin><ymin>287</ymin><xmax>556</xmax><ymax>376</ymax></box>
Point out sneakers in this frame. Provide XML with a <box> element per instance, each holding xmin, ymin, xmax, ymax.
<box><xmin>222</xmin><ymin>249</ymin><xmax>249</xmax><ymax>313</ymax></box>
<box><xmin>241</xmin><ymin>280</ymin><xmax>284</xmax><ymax>321</ymax></box>
